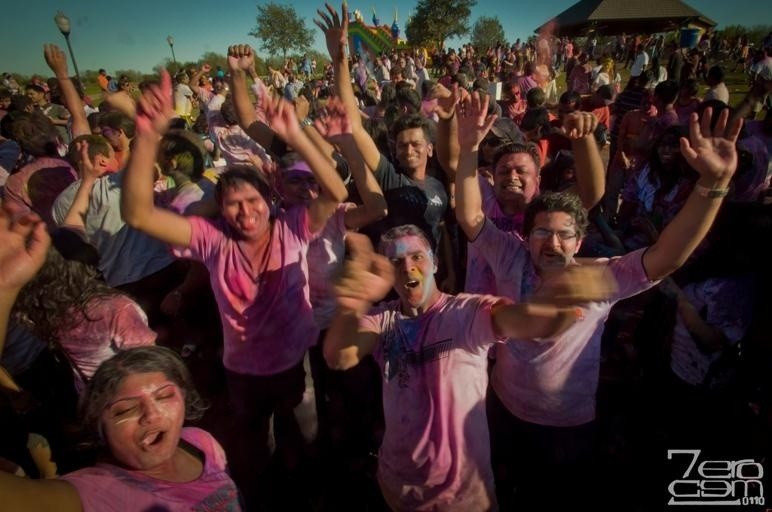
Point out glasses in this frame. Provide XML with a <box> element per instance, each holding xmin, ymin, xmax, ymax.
<box><xmin>481</xmin><ymin>138</ymin><xmax>512</xmax><ymax>148</ymax></box>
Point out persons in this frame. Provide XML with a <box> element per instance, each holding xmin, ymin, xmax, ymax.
<box><xmin>0</xmin><ymin>0</ymin><xmax>772</xmax><ymax>512</ymax></box>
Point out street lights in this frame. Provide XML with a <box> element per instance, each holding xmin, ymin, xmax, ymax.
<box><xmin>167</xmin><ymin>35</ymin><xmax>177</xmax><ymax>64</ymax></box>
<box><xmin>55</xmin><ymin>10</ymin><xmax>88</xmax><ymax>85</ymax></box>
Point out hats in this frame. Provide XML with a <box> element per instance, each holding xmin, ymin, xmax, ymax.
<box><xmin>491</xmin><ymin>117</ymin><xmax>523</xmax><ymax>144</ymax></box>
